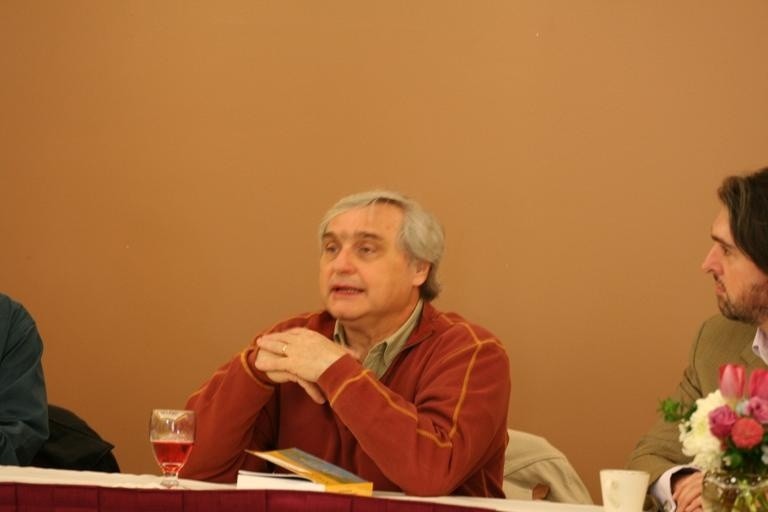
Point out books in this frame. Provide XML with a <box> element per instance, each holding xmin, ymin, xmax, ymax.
<box><xmin>235</xmin><ymin>445</ymin><xmax>374</xmax><ymax>497</ymax></box>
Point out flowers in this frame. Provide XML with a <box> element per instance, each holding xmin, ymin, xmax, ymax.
<box><xmin>655</xmin><ymin>362</ymin><xmax>768</xmax><ymax>512</ymax></box>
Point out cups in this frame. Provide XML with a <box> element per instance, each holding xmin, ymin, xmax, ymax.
<box><xmin>598</xmin><ymin>469</ymin><xmax>651</xmax><ymax>512</ymax></box>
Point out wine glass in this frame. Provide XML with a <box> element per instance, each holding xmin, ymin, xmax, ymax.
<box><xmin>149</xmin><ymin>408</ymin><xmax>196</xmax><ymax>488</ymax></box>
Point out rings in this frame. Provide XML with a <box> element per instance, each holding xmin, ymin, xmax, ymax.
<box><xmin>281</xmin><ymin>345</ymin><xmax>287</xmax><ymax>353</ymax></box>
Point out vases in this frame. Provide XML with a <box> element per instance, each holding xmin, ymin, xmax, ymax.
<box><xmin>700</xmin><ymin>468</ymin><xmax>768</xmax><ymax>512</ymax></box>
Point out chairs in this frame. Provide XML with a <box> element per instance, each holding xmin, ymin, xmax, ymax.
<box><xmin>503</xmin><ymin>429</ymin><xmax>594</xmax><ymax>504</ymax></box>
<box><xmin>31</xmin><ymin>405</ymin><xmax>120</xmax><ymax>474</ymax></box>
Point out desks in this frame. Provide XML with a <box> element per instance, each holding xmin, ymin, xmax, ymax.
<box><xmin>0</xmin><ymin>467</ymin><xmax>596</xmax><ymax>512</ymax></box>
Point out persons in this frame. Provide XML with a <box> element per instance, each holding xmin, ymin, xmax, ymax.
<box><xmin>626</xmin><ymin>166</ymin><xmax>767</xmax><ymax>512</ymax></box>
<box><xmin>0</xmin><ymin>294</ymin><xmax>52</xmax><ymax>466</ymax></box>
<box><xmin>180</xmin><ymin>192</ymin><xmax>510</xmax><ymax>502</ymax></box>
